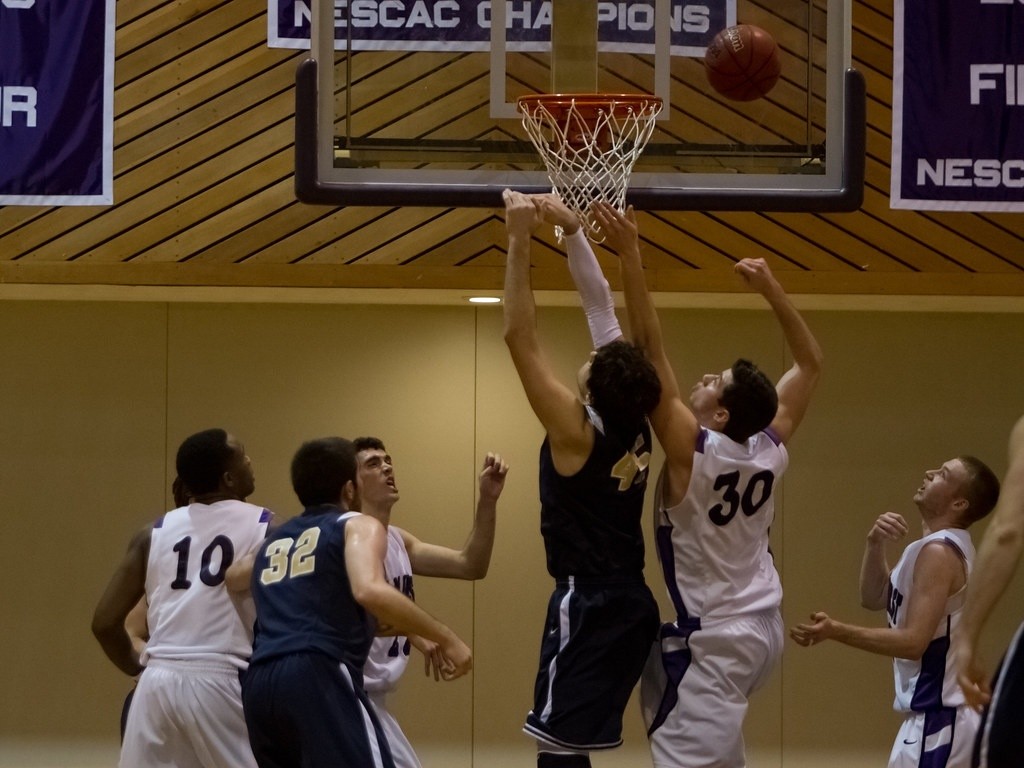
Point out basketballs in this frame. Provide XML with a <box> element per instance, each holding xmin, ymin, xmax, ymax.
<box><xmin>703</xmin><ymin>23</ymin><xmax>783</xmax><ymax>104</ymax></box>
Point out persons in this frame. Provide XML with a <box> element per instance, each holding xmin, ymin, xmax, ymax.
<box><xmin>91</xmin><ymin>427</ymin><xmax>288</xmax><ymax>768</ymax></box>
<box><xmin>499</xmin><ymin>183</ymin><xmax>670</xmax><ymax>768</ymax></box>
<box><xmin>223</xmin><ymin>434</ymin><xmax>512</xmax><ymax>768</ymax></box>
<box><xmin>952</xmin><ymin>417</ymin><xmax>1024</xmax><ymax>768</ymax></box>
<box><xmin>583</xmin><ymin>190</ymin><xmax>826</xmax><ymax>768</ymax></box>
<box><xmin>786</xmin><ymin>452</ymin><xmax>999</xmax><ymax>768</ymax></box>
